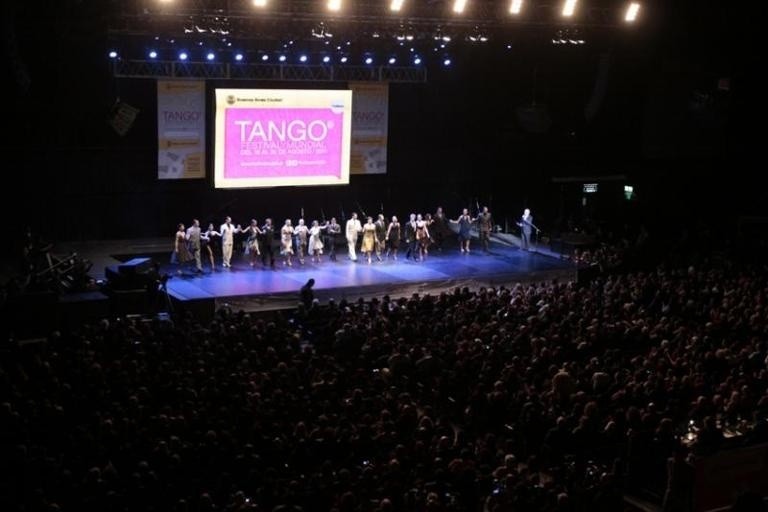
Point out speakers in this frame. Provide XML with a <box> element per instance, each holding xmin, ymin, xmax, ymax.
<box><xmin>56</xmin><ymin>292</ymin><xmax>108</xmax><ymax>327</ymax></box>
<box><xmin>0</xmin><ymin>290</ymin><xmax>56</xmax><ymax>342</ymax></box>
<box><xmin>112</xmin><ymin>289</ymin><xmax>148</xmax><ymax>313</ymax></box>
<box><xmin>574</xmin><ymin>263</ymin><xmax>599</xmax><ymax>283</ymax></box>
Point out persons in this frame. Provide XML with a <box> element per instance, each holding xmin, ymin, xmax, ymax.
<box><xmin>168</xmin><ymin>207</ymin><xmax>541</xmax><ymax>281</ymax></box>
<box><xmin>145</xmin><ymin>262</ymin><xmax>172</xmax><ymax>314</ymax></box>
<box><xmin>2</xmin><ymin>272</ymin><xmax>767</xmax><ymax>511</ymax></box>
<box><xmin>550</xmin><ymin>215</ymin><xmax>758</xmax><ymax>272</ymax></box>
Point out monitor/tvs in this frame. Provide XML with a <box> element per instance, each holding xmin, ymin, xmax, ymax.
<box><xmin>583</xmin><ymin>183</ymin><xmax>599</xmax><ymax>193</ymax></box>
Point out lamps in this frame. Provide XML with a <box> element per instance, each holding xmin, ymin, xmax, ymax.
<box><xmin>552</xmin><ymin>25</ymin><xmax>585</xmax><ymax>45</ymax></box>
<box><xmin>183</xmin><ymin>17</ymin><xmax>230</xmax><ymax>35</ymax></box>
<box><xmin>311</xmin><ymin>22</ymin><xmax>333</xmax><ymax>38</ymax></box>
<box><xmin>433</xmin><ymin>25</ymin><xmax>452</xmax><ymax>42</ymax></box>
<box><xmin>468</xmin><ymin>25</ymin><xmax>488</xmax><ymax>43</ymax></box>
<box><xmin>396</xmin><ymin>25</ymin><xmax>415</xmax><ymax>41</ymax></box>
